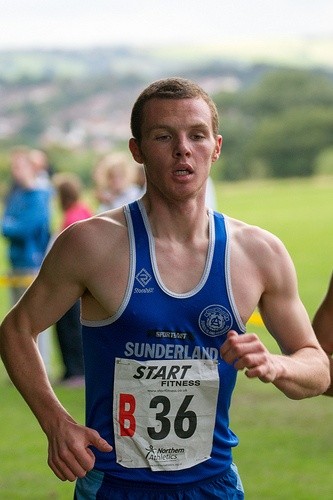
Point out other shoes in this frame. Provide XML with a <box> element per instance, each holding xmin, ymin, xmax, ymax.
<box><xmin>56</xmin><ymin>374</ymin><xmax>84</xmax><ymax>387</ymax></box>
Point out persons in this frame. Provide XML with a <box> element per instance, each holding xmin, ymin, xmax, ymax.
<box><xmin>49</xmin><ymin>179</ymin><xmax>94</xmax><ymax>388</ymax></box>
<box><xmin>311</xmin><ymin>278</ymin><xmax>332</xmax><ymax>399</ymax></box>
<box><xmin>95</xmin><ymin>149</ymin><xmax>144</xmax><ymax>212</ymax></box>
<box><xmin>2</xmin><ymin>79</ymin><xmax>331</xmax><ymax>500</ymax></box>
<box><xmin>2</xmin><ymin>146</ymin><xmax>53</xmax><ymax>383</ymax></box>
